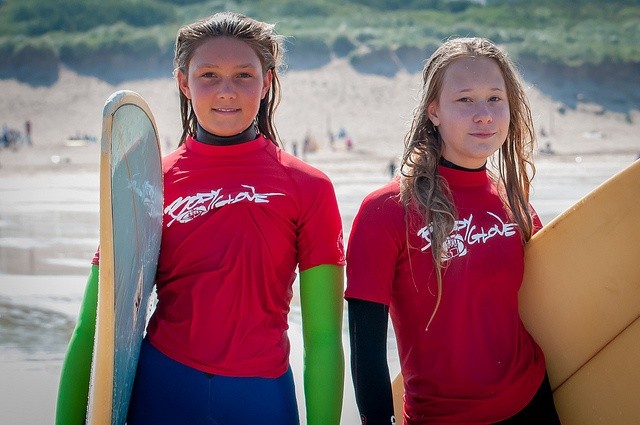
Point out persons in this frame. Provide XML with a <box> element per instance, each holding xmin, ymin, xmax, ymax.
<box><xmin>345</xmin><ymin>38</ymin><xmax>562</xmax><ymax>425</ymax></box>
<box><xmin>54</xmin><ymin>12</ymin><xmax>346</xmax><ymax>425</ymax></box>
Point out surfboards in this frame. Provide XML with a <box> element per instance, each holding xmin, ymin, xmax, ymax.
<box><xmin>85</xmin><ymin>90</ymin><xmax>162</xmax><ymax>423</ymax></box>
<box><xmin>393</xmin><ymin>160</ymin><xmax>639</xmax><ymax>424</ymax></box>
